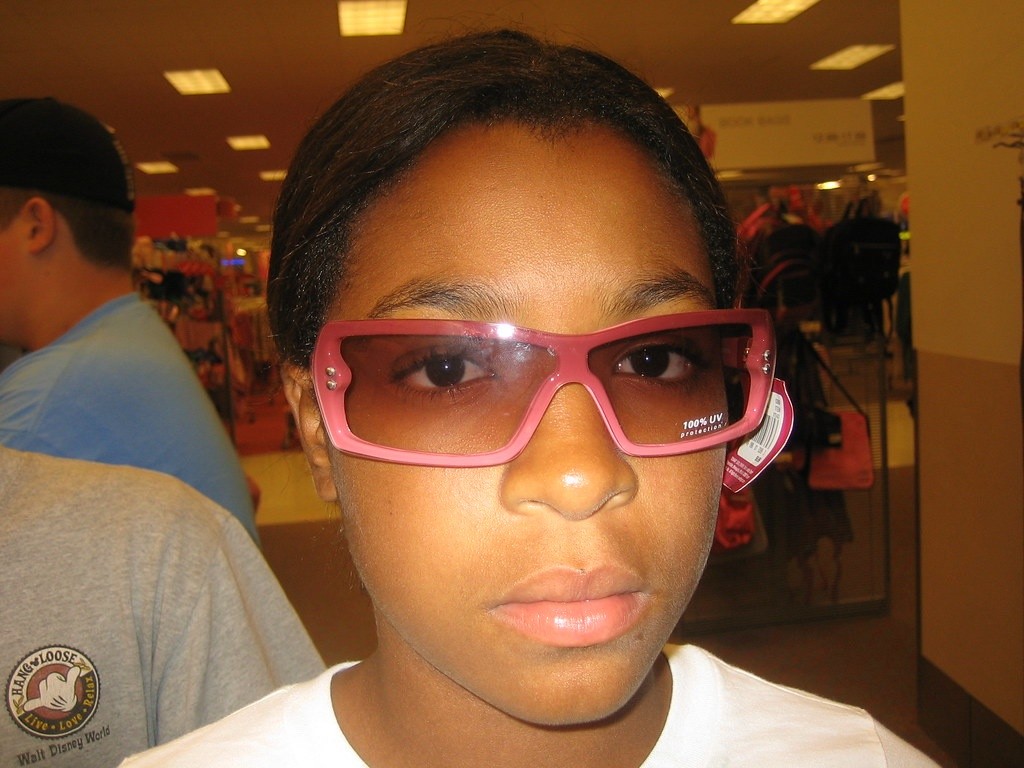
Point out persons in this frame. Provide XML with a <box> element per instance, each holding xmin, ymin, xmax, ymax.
<box><xmin>0</xmin><ymin>97</ymin><xmax>261</xmax><ymax>545</ymax></box>
<box><xmin>116</xmin><ymin>32</ymin><xmax>941</xmax><ymax>768</ymax></box>
<box><xmin>0</xmin><ymin>444</ymin><xmax>323</xmax><ymax>768</ymax></box>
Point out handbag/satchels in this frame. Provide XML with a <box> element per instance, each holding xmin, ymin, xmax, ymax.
<box><xmin>806</xmin><ymin>409</ymin><xmax>877</xmax><ymax>492</ymax></box>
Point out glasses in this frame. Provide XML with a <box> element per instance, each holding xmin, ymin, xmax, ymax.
<box><xmin>310</xmin><ymin>309</ymin><xmax>777</xmax><ymax>468</ymax></box>
